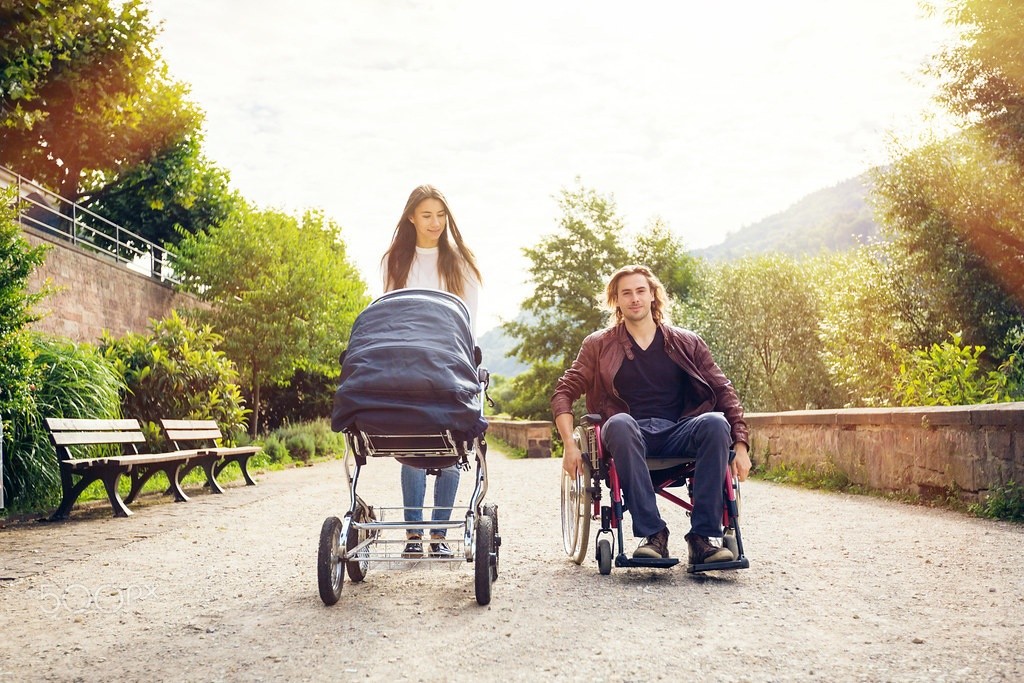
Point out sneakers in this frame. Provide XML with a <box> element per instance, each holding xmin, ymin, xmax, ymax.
<box><xmin>633</xmin><ymin>527</ymin><xmax>670</xmax><ymax>558</ymax></box>
<box><xmin>684</xmin><ymin>532</ymin><xmax>734</xmax><ymax>564</ymax></box>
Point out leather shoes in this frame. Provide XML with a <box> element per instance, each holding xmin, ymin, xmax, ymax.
<box><xmin>401</xmin><ymin>536</ymin><xmax>423</xmax><ymax>558</ymax></box>
<box><xmin>428</xmin><ymin>537</ymin><xmax>454</xmax><ymax>558</ymax></box>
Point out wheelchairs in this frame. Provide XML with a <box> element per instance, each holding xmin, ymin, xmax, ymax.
<box><xmin>560</xmin><ymin>414</ymin><xmax>749</xmax><ymax>574</ymax></box>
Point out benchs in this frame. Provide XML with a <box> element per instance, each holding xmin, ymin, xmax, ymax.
<box><xmin>157</xmin><ymin>418</ymin><xmax>262</xmax><ymax>496</ymax></box>
<box><xmin>43</xmin><ymin>418</ymin><xmax>197</xmax><ymax>522</ymax></box>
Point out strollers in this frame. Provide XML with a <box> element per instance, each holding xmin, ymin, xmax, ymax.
<box><xmin>317</xmin><ymin>288</ymin><xmax>501</xmax><ymax>607</ymax></box>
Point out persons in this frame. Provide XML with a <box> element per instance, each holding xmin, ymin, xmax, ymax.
<box><xmin>551</xmin><ymin>264</ymin><xmax>752</xmax><ymax>561</ymax></box>
<box><xmin>384</xmin><ymin>185</ymin><xmax>481</xmax><ymax>557</ymax></box>
